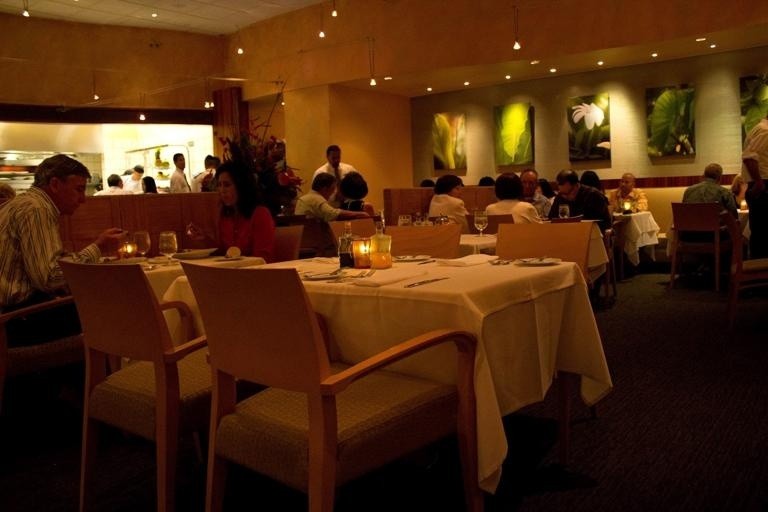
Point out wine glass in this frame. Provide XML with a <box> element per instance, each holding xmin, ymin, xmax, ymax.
<box><xmin>133</xmin><ymin>232</ymin><xmax>152</xmax><ymax>264</ymax></box>
<box><xmin>472</xmin><ymin>210</ymin><xmax>490</xmax><ymax>238</ymax></box>
<box><xmin>158</xmin><ymin>233</ymin><xmax>179</xmax><ymax>267</ymax></box>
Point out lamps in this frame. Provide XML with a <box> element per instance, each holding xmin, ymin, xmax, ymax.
<box><xmin>331</xmin><ymin>0</ymin><xmax>337</xmax><ymax>17</ymax></box>
<box><xmin>513</xmin><ymin>6</ymin><xmax>521</xmax><ymax>50</ymax></box>
<box><xmin>237</xmin><ymin>34</ymin><xmax>243</xmax><ymax>54</ymax></box>
<box><xmin>366</xmin><ymin>37</ymin><xmax>376</xmax><ymax>86</ymax></box>
<box><xmin>319</xmin><ymin>6</ymin><xmax>325</xmax><ymax>38</ymax></box>
<box><xmin>139</xmin><ymin>93</ymin><xmax>145</xmax><ymax>120</ymax></box>
<box><xmin>93</xmin><ymin>76</ymin><xmax>100</xmax><ymax>101</ymax></box>
<box><xmin>22</xmin><ymin>0</ymin><xmax>30</xmax><ymax>18</ymax></box>
<box><xmin>204</xmin><ymin>79</ymin><xmax>214</xmax><ymax>108</ymax></box>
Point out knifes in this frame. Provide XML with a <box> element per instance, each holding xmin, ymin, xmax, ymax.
<box><xmin>405</xmin><ymin>277</ymin><xmax>451</xmax><ymax>288</ymax></box>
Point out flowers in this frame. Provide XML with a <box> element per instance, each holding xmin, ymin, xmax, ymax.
<box><xmin>200</xmin><ymin>114</ymin><xmax>308</xmax><ymax>218</ymax></box>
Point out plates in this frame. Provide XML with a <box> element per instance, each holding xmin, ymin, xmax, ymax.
<box><xmin>300</xmin><ymin>273</ymin><xmax>344</xmax><ymax>280</ymax></box>
<box><xmin>392</xmin><ymin>256</ymin><xmax>431</xmax><ymax>262</ymax></box>
<box><xmin>176</xmin><ymin>248</ymin><xmax>219</xmax><ymax>258</ymax></box>
<box><xmin>512</xmin><ymin>258</ymin><xmax>562</xmax><ymax>265</ymax></box>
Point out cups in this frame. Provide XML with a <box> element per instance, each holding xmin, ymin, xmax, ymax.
<box><xmin>558</xmin><ymin>204</ymin><xmax>570</xmax><ymax>219</ymax></box>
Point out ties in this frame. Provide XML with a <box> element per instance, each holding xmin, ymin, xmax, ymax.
<box><xmin>335</xmin><ymin>167</ymin><xmax>340</xmax><ymax>182</ymax></box>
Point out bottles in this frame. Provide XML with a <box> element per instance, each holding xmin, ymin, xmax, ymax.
<box><xmin>335</xmin><ymin>222</ymin><xmax>393</xmax><ymax>268</ymax></box>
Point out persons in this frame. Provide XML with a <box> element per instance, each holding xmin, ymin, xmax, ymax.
<box><xmin>170</xmin><ymin>153</ymin><xmax>220</xmax><ymax>193</ymax></box>
<box><xmin>311</xmin><ymin>144</ymin><xmax>356</xmax><ymax>208</ymax></box>
<box><xmin>1</xmin><ymin>154</ymin><xmax>129</xmax><ymax>348</ymax></box>
<box><xmin>92</xmin><ymin>173</ymin><xmax>134</xmax><ymax>196</ymax></box>
<box><xmin>120</xmin><ymin>164</ymin><xmax>158</xmax><ymax>194</ymax></box>
<box><xmin>419</xmin><ymin>169</ymin><xmax>648</xmax><ymax>306</ymax></box>
<box><xmin>295</xmin><ymin>172</ymin><xmax>370</xmax><ymax>221</ymax></box>
<box><xmin>338</xmin><ymin>171</ymin><xmax>385</xmax><ymax>267</ymax></box>
<box><xmin>680</xmin><ymin>157</ymin><xmax>768</xmax><ymax>296</ymax></box>
<box><xmin>213</xmin><ymin>162</ymin><xmax>295</xmax><ymax>264</ymax></box>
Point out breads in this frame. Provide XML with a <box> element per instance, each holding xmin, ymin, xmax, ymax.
<box><xmin>226</xmin><ymin>246</ymin><xmax>241</xmax><ymax>259</ymax></box>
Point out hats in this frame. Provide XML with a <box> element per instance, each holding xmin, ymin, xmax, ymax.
<box><xmin>339</xmin><ymin>171</ymin><xmax>368</xmax><ymax>200</ymax></box>
